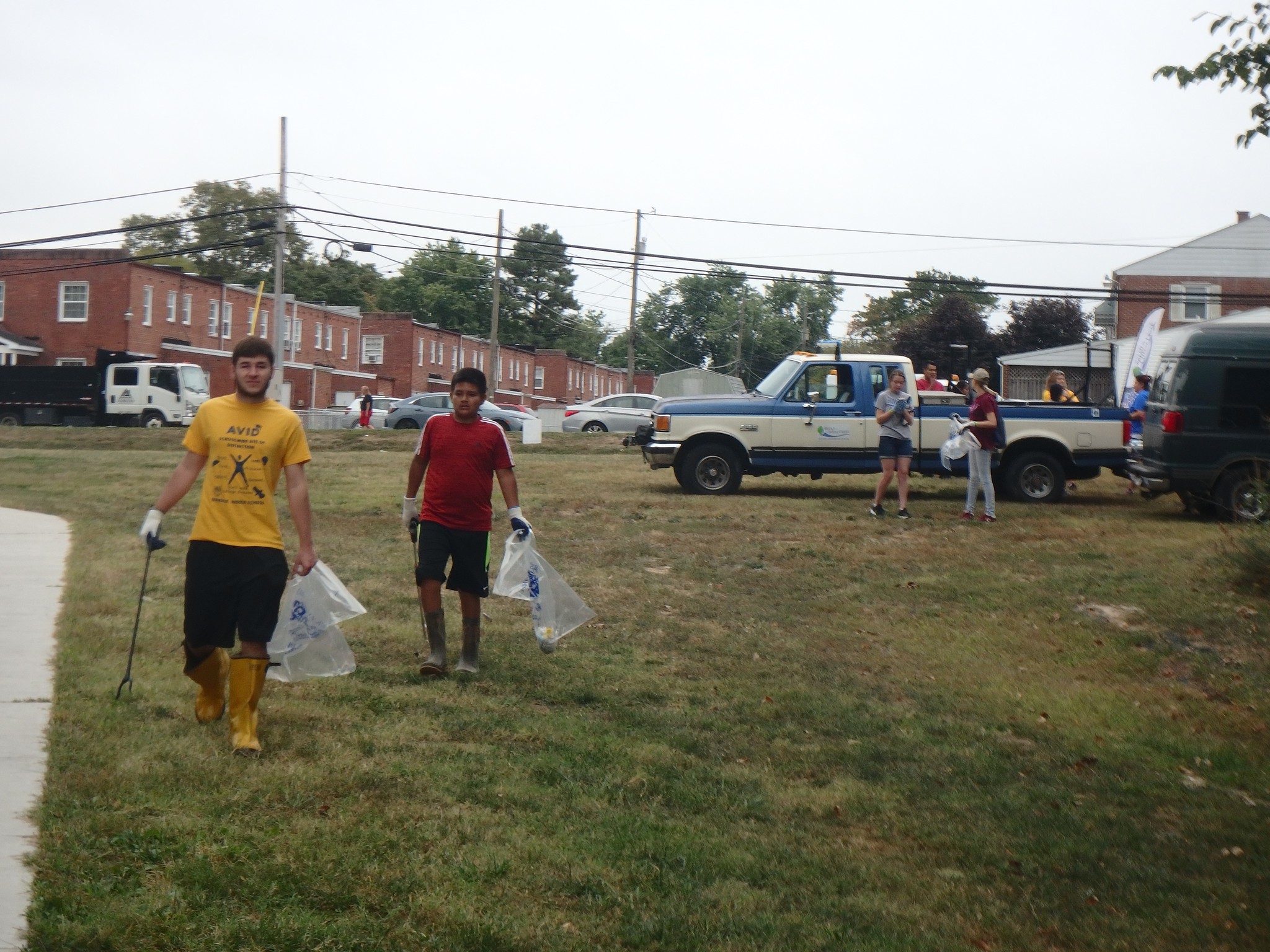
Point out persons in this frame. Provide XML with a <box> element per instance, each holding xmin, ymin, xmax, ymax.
<box><xmin>915</xmin><ymin>360</ymin><xmax>945</xmax><ymax>391</ymax></box>
<box><xmin>360</xmin><ymin>386</ymin><xmax>377</xmax><ymax>436</ymax></box>
<box><xmin>948</xmin><ymin>368</ymin><xmax>999</xmax><ymax>522</ymax></box>
<box><xmin>869</xmin><ymin>368</ymin><xmax>915</xmax><ymax>519</ymax></box>
<box><xmin>139</xmin><ymin>340</ymin><xmax>320</xmax><ymax>761</ymax></box>
<box><xmin>402</xmin><ymin>366</ymin><xmax>533</xmax><ymax>677</ymax></box>
<box><xmin>1128</xmin><ymin>375</ymin><xmax>1152</xmax><ymax>493</ymax></box>
<box><xmin>1042</xmin><ymin>370</ymin><xmax>1079</xmax><ymax>490</ymax></box>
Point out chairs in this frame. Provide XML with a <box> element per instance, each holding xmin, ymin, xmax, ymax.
<box><xmin>835</xmin><ymin>382</ymin><xmax>854</xmax><ymax>403</ymax></box>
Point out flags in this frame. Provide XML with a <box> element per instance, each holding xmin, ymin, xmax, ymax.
<box><xmin>1120</xmin><ymin>306</ymin><xmax>1165</xmax><ymax>409</ymax></box>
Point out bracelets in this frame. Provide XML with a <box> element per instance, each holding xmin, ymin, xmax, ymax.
<box><xmin>889</xmin><ymin>409</ymin><xmax>894</xmax><ymax>415</ymax></box>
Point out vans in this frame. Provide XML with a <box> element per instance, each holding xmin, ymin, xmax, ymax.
<box><xmin>1123</xmin><ymin>323</ymin><xmax>1270</xmax><ymax>527</ymax></box>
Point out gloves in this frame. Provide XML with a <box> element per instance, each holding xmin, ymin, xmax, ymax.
<box><xmin>402</xmin><ymin>494</ymin><xmax>418</xmax><ymax>529</ymax></box>
<box><xmin>949</xmin><ymin>412</ymin><xmax>976</xmax><ymax>435</ymax></box>
<box><xmin>893</xmin><ymin>399</ymin><xmax>906</xmax><ymax>414</ymax></box>
<box><xmin>140</xmin><ymin>509</ymin><xmax>166</xmax><ymax>551</ymax></box>
<box><xmin>507</xmin><ymin>506</ymin><xmax>533</xmax><ymax>542</ymax></box>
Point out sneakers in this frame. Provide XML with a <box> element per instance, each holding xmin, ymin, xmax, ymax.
<box><xmin>870</xmin><ymin>503</ymin><xmax>886</xmax><ymax>516</ymax></box>
<box><xmin>978</xmin><ymin>515</ymin><xmax>996</xmax><ymax>523</ymax></box>
<box><xmin>897</xmin><ymin>508</ymin><xmax>910</xmax><ymax>519</ymax></box>
<box><xmin>963</xmin><ymin>513</ymin><xmax>973</xmax><ymax>520</ymax></box>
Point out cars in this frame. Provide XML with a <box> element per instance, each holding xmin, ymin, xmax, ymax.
<box><xmin>493</xmin><ymin>402</ymin><xmax>538</xmax><ymax>419</ymax></box>
<box><xmin>562</xmin><ymin>393</ymin><xmax>664</xmax><ymax>433</ymax></box>
<box><xmin>384</xmin><ymin>392</ymin><xmax>538</xmax><ymax>431</ymax></box>
<box><xmin>343</xmin><ymin>394</ymin><xmax>405</xmax><ymax>430</ymax></box>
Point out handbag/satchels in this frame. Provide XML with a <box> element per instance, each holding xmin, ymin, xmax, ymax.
<box><xmin>492</xmin><ymin>529</ymin><xmax>598</xmax><ymax>654</ymax></box>
<box><xmin>941</xmin><ymin>417</ymin><xmax>982</xmax><ymax>472</ymax></box>
<box><xmin>265</xmin><ymin>559</ymin><xmax>368</xmax><ymax>685</ymax></box>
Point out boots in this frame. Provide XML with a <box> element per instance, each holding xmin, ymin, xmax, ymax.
<box><xmin>455</xmin><ymin>617</ymin><xmax>480</xmax><ymax>675</ymax></box>
<box><xmin>228</xmin><ymin>652</ymin><xmax>282</xmax><ymax>755</ymax></box>
<box><xmin>420</xmin><ymin>609</ymin><xmax>450</xmax><ymax>675</ymax></box>
<box><xmin>183</xmin><ymin>646</ymin><xmax>231</xmax><ymax>727</ymax></box>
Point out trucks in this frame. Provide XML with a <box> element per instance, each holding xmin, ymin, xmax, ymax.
<box><xmin>0</xmin><ymin>348</ymin><xmax>211</xmax><ymax>428</ymax></box>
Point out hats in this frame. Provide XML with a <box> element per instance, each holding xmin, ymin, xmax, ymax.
<box><xmin>967</xmin><ymin>368</ymin><xmax>990</xmax><ymax>384</ymax></box>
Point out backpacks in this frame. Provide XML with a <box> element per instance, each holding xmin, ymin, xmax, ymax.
<box><xmin>990</xmin><ymin>405</ymin><xmax>1006</xmax><ymax>449</ymax></box>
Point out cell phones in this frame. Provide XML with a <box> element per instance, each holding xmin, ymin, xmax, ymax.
<box><xmin>899</xmin><ymin>398</ymin><xmax>906</xmax><ymax>400</ymax></box>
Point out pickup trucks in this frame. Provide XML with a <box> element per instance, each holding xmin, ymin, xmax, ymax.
<box><xmin>644</xmin><ymin>342</ymin><xmax>1132</xmax><ymax>504</ymax></box>
